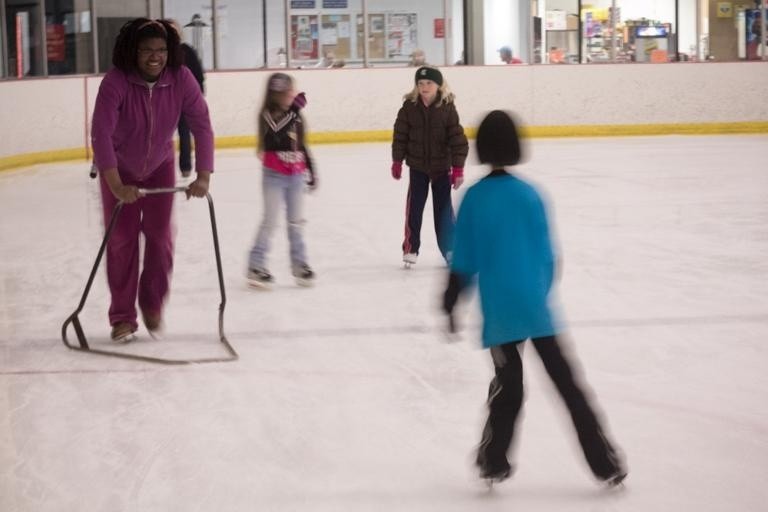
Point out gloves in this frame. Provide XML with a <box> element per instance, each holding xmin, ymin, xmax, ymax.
<box><xmin>291</xmin><ymin>92</ymin><xmax>306</xmax><ymax>110</ymax></box>
<box><xmin>392</xmin><ymin>161</ymin><xmax>402</xmax><ymax>179</ymax></box>
<box><xmin>450</xmin><ymin>167</ymin><xmax>463</xmax><ymax>189</ymax></box>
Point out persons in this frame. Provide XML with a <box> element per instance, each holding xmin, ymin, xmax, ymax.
<box><xmin>166</xmin><ymin>19</ymin><xmax>204</xmax><ymax>177</ymax></box>
<box><xmin>496</xmin><ymin>46</ymin><xmax>523</xmax><ymax>64</ymax></box>
<box><xmin>247</xmin><ymin>73</ymin><xmax>318</xmax><ymax>283</ymax></box>
<box><xmin>408</xmin><ymin>50</ymin><xmax>426</xmax><ymax>66</ymax></box>
<box><xmin>316</xmin><ymin>49</ymin><xmax>346</xmax><ymax>68</ymax></box>
<box><xmin>91</xmin><ymin>17</ymin><xmax>214</xmax><ymax>341</ymax></box>
<box><xmin>442</xmin><ymin>109</ymin><xmax>629</xmax><ymax>486</ymax></box>
<box><xmin>390</xmin><ymin>66</ymin><xmax>469</xmax><ymax>263</ymax></box>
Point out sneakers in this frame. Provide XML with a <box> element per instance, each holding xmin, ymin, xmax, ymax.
<box><xmin>292</xmin><ymin>266</ymin><xmax>314</xmax><ymax>279</ymax></box>
<box><xmin>144</xmin><ymin>314</ymin><xmax>161</xmax><ymax>332</ymax></box>
<box><xmin>247</xmin><ymin>269</ymin><xmax>271</xmax><ymax>281</ymax></box>
<box><xmin>110</xmin><ymin>320</ymin><xmax>137</xmax><ymax>340</ymax></box>
<box><xmin>403</xmin><ymin>251</ymin><xmax>418</xmax><ymax>264</ymax></box>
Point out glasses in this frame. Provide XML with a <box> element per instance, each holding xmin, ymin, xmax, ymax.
<box><xmin>135</xmin><ymin>48</ymin><xmax>169</xmax><ymax>57</ymax></box>
